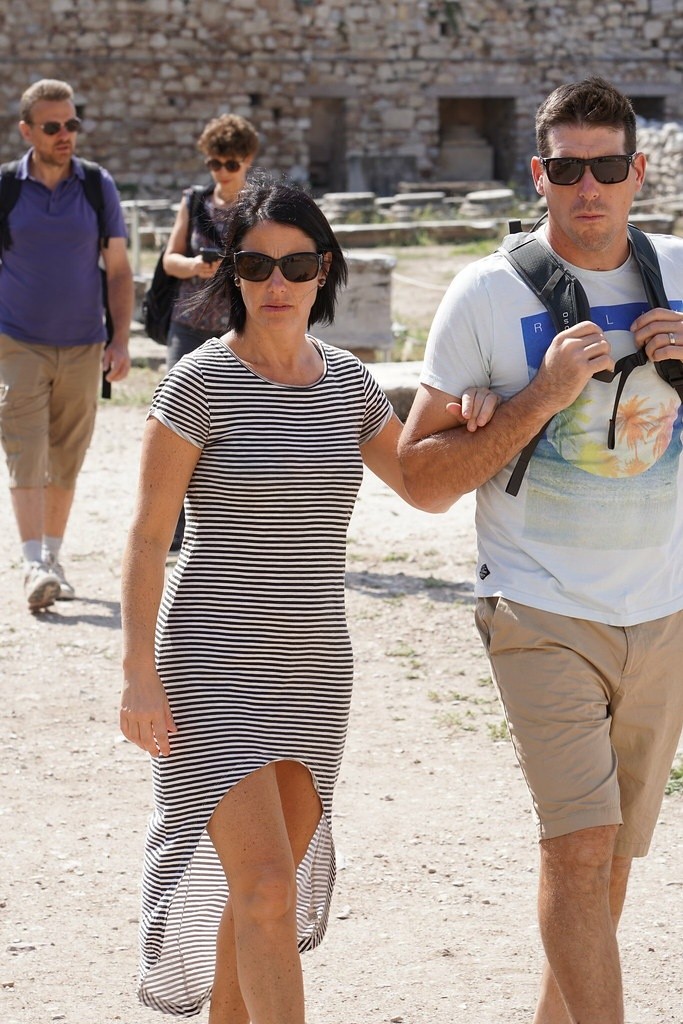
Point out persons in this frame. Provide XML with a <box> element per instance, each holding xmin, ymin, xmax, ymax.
<box><xmin>116</xmin><ymin>180</ymin><xmax>506</xmax><ymax>1024</ymax></box>
<box><xmin>0</xmin><ymin>80</ymin><xmax>134</xmax><ymax>612</ymax></box>
<box><xmin>158</xmin><ymin>114</ymin><xmax>266</xmax><ymax>564</ymax></box>
<box><xmin>395</xmin><ymin>77</ymin><xmax>681</xmax><ymax>1022</ymax></box>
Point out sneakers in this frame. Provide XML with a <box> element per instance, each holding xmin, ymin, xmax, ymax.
<box><xmin>21</xmin><ymin>562</ymin><xmax>60</xmax><ymax>611</ymax></box>
<box><xmin>47</xmin><ymin>561</ymin><xmax>77</xmax><ymax>601</ymax></box>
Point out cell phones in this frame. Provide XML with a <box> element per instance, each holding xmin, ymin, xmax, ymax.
<box><xmin>200</xmin><ymin>247</ymin><xmax>221</xmax><ymax>263</ymax></box>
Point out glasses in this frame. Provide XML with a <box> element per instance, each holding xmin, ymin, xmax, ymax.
<box><xmin>203</xmin><ymin>158</ymin><xmax>244</xmax><ymax>173</ymax></box>
<box><xmin>538</xmin><ymin>152</ymin><xmax>638</xmax><ymax>185</ymax></box>
<box><xmin>231</xmin><ymin>249</ymin><xmax>325</xmax><ymax>284</ymax></box>
<box><xmin>29</xmin><ymin>118</ymin><xmax>83</xmax><ymax>136</ymax></box>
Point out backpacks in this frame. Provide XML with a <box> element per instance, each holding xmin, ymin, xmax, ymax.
<box><xmin>140</xmin><ymin>182</ymin><xmax>209</xmax><ymax>349</ymax></box>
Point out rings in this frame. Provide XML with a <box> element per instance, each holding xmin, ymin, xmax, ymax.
<box><xmin>668</xmin><ymin>333</ymin><xmax>675</xmax><ymax>347</ymax></box>
